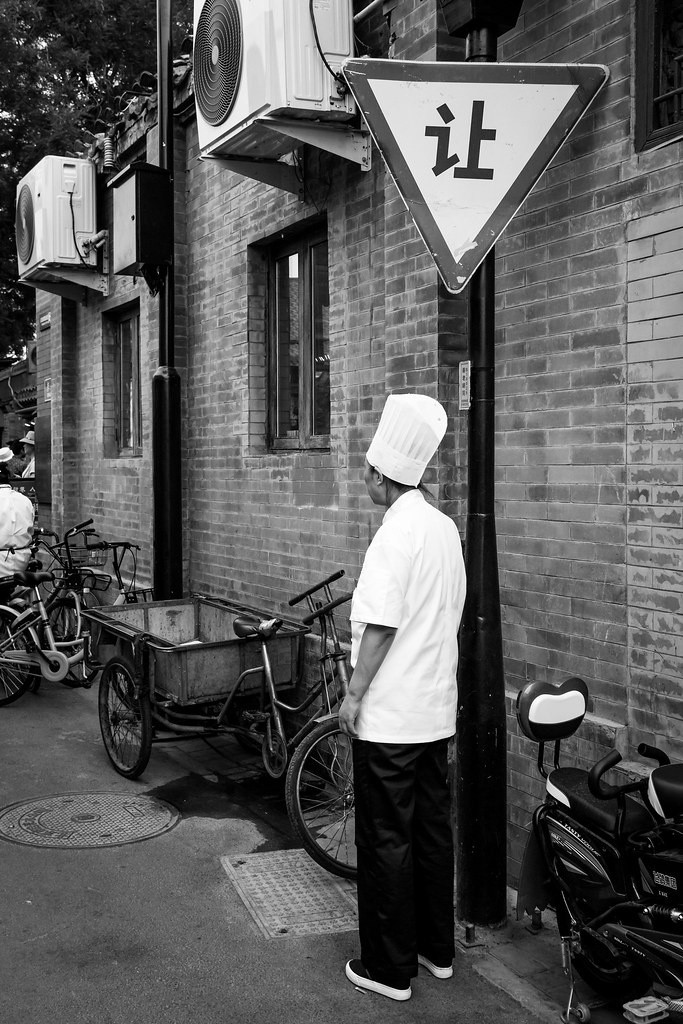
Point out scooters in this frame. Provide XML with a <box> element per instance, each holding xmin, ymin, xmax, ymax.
<box><xmin>512</xmin><ymin>674</ymin><xmax>683</xmax><ymax>1024</ymax></box>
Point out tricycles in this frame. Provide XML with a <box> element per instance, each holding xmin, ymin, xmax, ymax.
<box><xmin>80</xmin><ymin>568</ymin><xmax>356</xmax><ymax>879</ymax></box>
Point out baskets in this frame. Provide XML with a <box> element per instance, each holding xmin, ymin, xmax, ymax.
<box><xmin>59</xmin><ymin>546</ymin><xmax>108</xmax><ymax>569</ymax></box>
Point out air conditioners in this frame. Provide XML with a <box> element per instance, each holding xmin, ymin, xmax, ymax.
<box><xmin>14</xmin><ymin>154</ymin><xmax>106</xmax><ymax>280</ymax></box>
<box><xmin>191</xmin><ymin>0</ymin><xmax>355</xmax><ymax>160</ymax></box>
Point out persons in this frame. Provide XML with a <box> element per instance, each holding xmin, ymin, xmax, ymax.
<box><xmin>19</xmin><ymin>431</ymin><xmax>35</xmax><ymax>478</ymax></box>
<box><xmin>0</xmin><ymin>471</ymin><xmax>34</xmax><ymax>578</ymax></box>
<box><xmin>338</xmin><ymin>393</ymin><xmax>467</xmax><ymax>1001</ymax></box>
<box><xmin>0</xmin><ymin>446</ymin><xmax>14</xmax><ymax>473</ymax></box>
<box><xmin>6</xmin><ymin>438</ymin><xmax>27</xmax><ymax>478</ymax></box>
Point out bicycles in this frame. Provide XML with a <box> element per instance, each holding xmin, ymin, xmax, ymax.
<box><xmin>0</xmin><ymin>519</ymin><xmax>138</xmax><ymax>708</ymax></box>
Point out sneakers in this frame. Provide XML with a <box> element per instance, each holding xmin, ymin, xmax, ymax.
<box><xmin>345</xmin><ymin>958</ymin><xmax>412</xmax><ymax>1001</ymax></box>
<box><xmin>418</xmin><ymin>953</ymin><xmax>452</xmax><ymax>979</ymax></box>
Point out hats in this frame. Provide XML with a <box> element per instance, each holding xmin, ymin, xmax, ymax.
<box><xmin>365</xmin><ymin>393</ymin><xmax>448</xmax><ymax>486</ymax></box>
<box><xmin>0</xmin><ymin>446</ymin><xmax>14</xmax><ymax>462</ymax></box>
<box><xmin>19</xmin><ymin>430</ymin><xmax>35</xmax><ymax>445</ymax></box>
